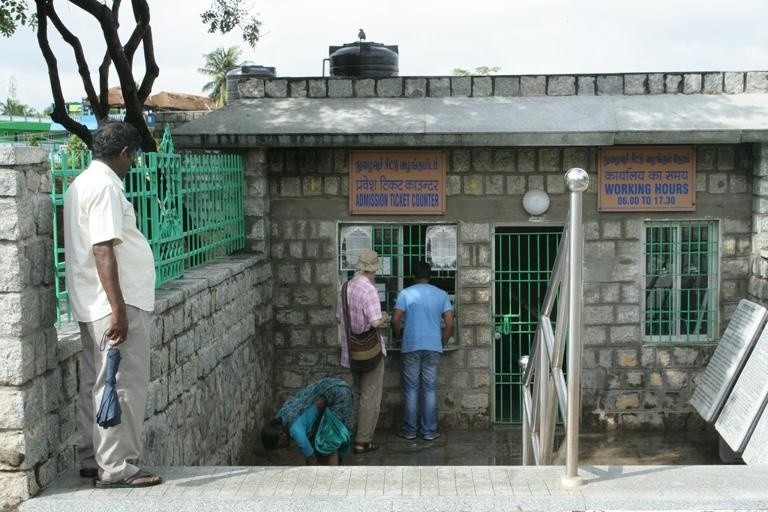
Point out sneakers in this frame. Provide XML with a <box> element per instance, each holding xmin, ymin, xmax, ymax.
<box><xmin>399</xmin><ymin>431</ymin><xmax>416</xmax><ymax>440</ymax></box>
<box><xmin>423</xmin><ymin>432</ymin><xmax>440</xmax><ymax>440</ymax></box>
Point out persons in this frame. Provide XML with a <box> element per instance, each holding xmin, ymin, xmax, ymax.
<box><xmin>389</xmin><ymin>260</ymin><xmax>454</xmax><ymax>440</ymax></box>
<box><xmin>62</xmin><ymin>118</ymin><xmax>163</xmax><ymax>491</ymax></box>
<box><xmin>260</xmin><ymin>376</ymin><xmax>355</xmax><ymax>466</ymax></box>
<box><xmin>335</xmin><ymin>249</ymin><xmax>389</xmax><ymax>453</ymax></box>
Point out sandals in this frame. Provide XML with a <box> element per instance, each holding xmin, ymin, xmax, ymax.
<box><xmin>353</xmin><ymin>441</ymin><xmax>378</xmax><ymax>453</ymax></box>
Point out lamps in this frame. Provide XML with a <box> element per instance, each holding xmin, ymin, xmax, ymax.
<box><xmin>522</xmin><ymin>188</ymin><xmax>550</xmax><ymax>223</ymax></box>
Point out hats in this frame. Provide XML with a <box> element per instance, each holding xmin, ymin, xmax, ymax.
<box><xmin>352</xmin><ymin>250</ymin><xmax>381</xmax><ymax>272</ymax></box>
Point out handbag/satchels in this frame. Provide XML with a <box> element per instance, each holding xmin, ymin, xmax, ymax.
<box><xmin>346</xmin><ymin>328</ymin><xmax>383</xmax><ymax>375</ymax></box>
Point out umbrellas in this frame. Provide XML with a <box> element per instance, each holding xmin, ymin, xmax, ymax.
<box><xmin>94</xmin><ymin>331</ymin><xmax>124</xmax><ymax>429</ymax></box>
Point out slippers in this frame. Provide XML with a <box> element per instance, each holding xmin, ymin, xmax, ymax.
<box><xmin>80</xmin><ymin>467</ymin><xmax>161</xmax><ymax>489</ymax></box>
